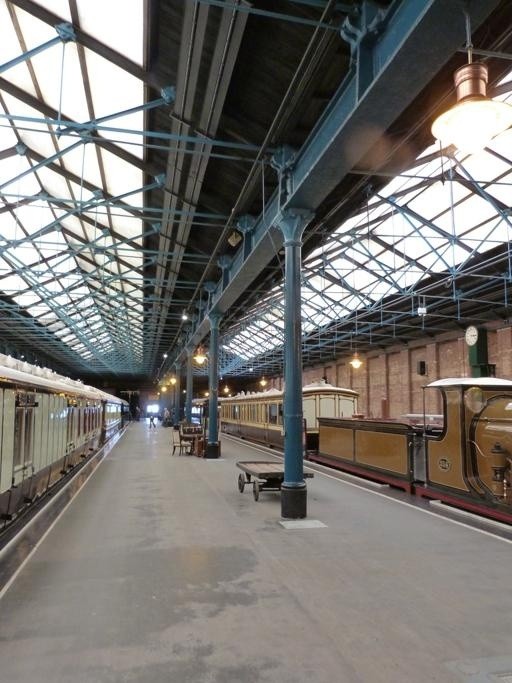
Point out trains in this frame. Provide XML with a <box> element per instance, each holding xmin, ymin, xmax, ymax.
<box><xmin>1</xmin><ymin>352</ymin><xmax>132</xmax><ymax>535</ymax></box>
<box><xmin>192</xmin><ymin>376</ymin><xmax>512</xmax><ymax>525</ymax></box>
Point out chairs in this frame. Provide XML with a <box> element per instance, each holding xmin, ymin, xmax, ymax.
<box><xmin>172</xmin><ymin>431</ymin><xmax>188</xmax><ymax>456</ymax></box>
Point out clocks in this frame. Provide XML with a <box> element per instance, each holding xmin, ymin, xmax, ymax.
<box><xmin>464</xmin><ymin>325</ymin><xmax>479</xmax><ymax>346</ymax></box>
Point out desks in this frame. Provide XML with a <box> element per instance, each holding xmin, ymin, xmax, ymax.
<box><xmin>178</xmin><ymin>423</ymin><xmax>203</xmax><ymax>455</ymax></box>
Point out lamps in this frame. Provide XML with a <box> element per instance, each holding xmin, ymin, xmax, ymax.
<box><xmin>259</xmin><ymin>370</ymin><xmax>268</xmax><ymax>386</ymax></box>
<box><xmin>430</xmin><ymin>47</ymin><xmax>512</xmax><ymax>147</ymax></box>
<box><xmin>192</xmin><ymin>343</ymin><xmax>206</xmax><ymax>364</ymax></box>
<box><xmin>348</xmin><ymin>343</ymin><xmax>363</xmax><ymax>369</ymax></box>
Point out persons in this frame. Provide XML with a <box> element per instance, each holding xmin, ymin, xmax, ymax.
<box><xmin>163</xmin><ymin>407</ymin><xmax>170</xmax><ymax>423</ymax></box>
<box><xmin>149</xmin><ymin>415</ymin><xmax>156</xmax><ymax>429</ymax></box>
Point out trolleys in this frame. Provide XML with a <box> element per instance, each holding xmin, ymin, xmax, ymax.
<box><xmin>236</xmin><ymin>459</ymin><xmax>314</xmax><ymax>501</ymax></box>
